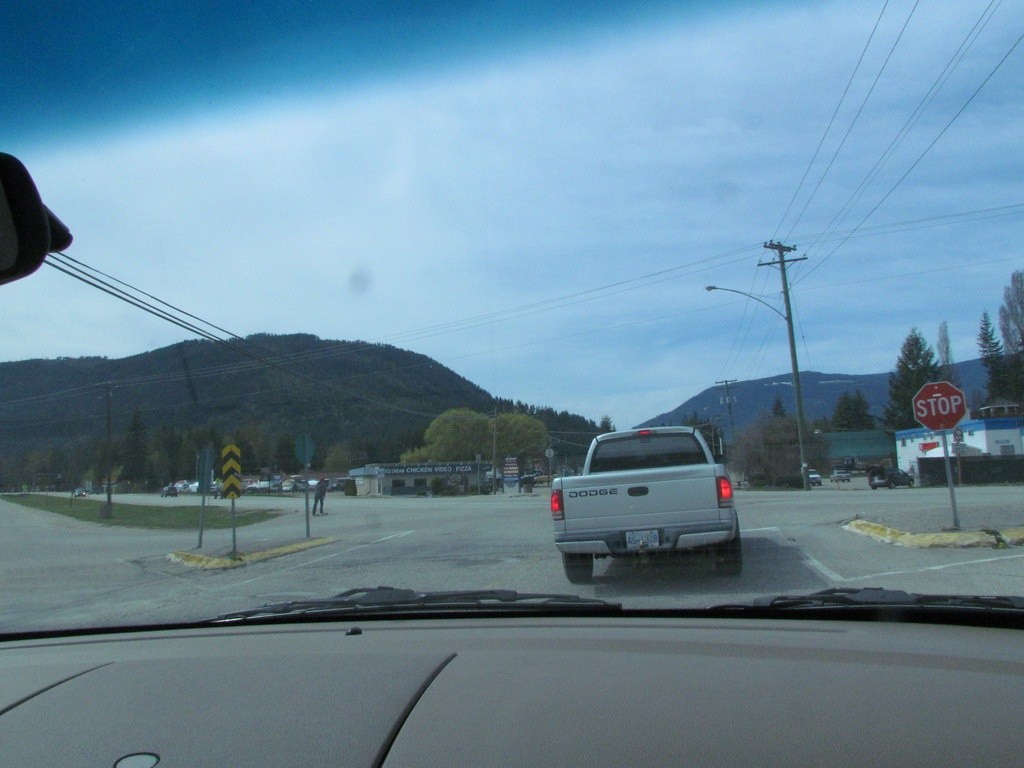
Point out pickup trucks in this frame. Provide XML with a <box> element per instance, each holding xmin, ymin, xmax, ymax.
<box><xmin>552</xmin><ymin>426</ymin><xmax>741</xmax><ymax>585</ymax></box>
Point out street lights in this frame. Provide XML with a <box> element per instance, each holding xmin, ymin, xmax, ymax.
<box><xmin>706</xmin><ymin>285</ymin><xmax>812</xmax><ymax>493</ymax></box>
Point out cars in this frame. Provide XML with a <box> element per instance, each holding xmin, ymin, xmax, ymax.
<box><xmin>174</xmin><ymin>472</ymin><xmax>353</xmax><ymax>492</ymax></box>
<box><xmin>828</xmin><ymin>469</ymin><xmax>851</xmax><ymax>483</ymax></box>
<box><xmin>73</xmin><ymin>487</ymin><xmax>86</xmax><ymax>498</ymax></box>
<box><xmin>868</xmin><ymin>467</ymin><xmax>914</xmax><ymax>490</ymax></box>
<box><xmin>808</xmin><ymin>469</ymin><xmax>822</xmax><ymax>487</ymax></box>
<box><xmin>160</xmin><ymin>486</ymin><xmax>178</xmax><ymax>498</ymax></box>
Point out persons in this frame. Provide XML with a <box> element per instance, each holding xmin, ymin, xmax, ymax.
<box><xmin>311</xmin><ymin>476</ymin><xmax>327</xmax><ymax>515</ymax></box>
